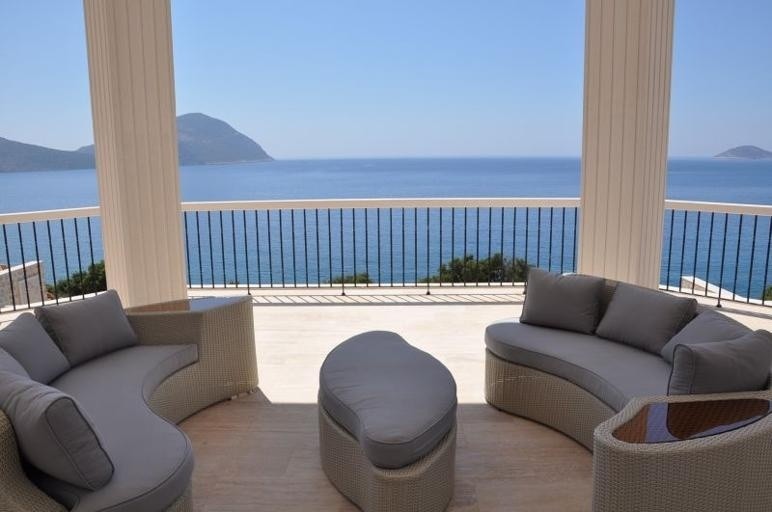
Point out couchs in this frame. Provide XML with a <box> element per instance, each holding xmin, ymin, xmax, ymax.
<box><xmin>0</xmin><ymin>288</ymin><xmax>260</xmax><ymax>511</ymax></box>
<box><xmin>483</xmin><ymin>265</ymin><xmax>772</xmax><ymax>511</ymax></box>
